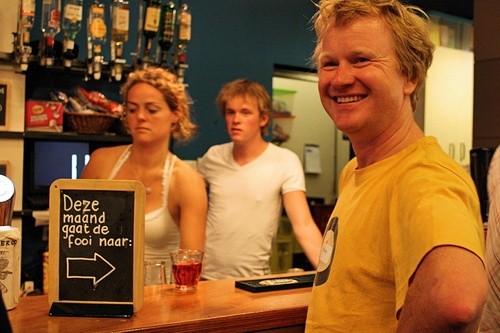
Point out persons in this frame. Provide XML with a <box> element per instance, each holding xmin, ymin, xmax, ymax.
<box><xmin>304</xmin><ymin>0</ymin><xmax>488</xmax><ymax>333</ymax></box>
<box><xmin>79</xmin><ymin>68</ymin><xmax>208</xmax><ymax>286</ymax></box>
<box><xmin>192</xmin><ymin>79</ymin><xmax>323</xmax><ymax>281</ymax></box>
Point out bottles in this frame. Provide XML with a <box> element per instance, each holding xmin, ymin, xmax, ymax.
<box><xmin>15</xmin><ymin>0</ymin><xmax>192</xmax><ymax>85</ymax></box>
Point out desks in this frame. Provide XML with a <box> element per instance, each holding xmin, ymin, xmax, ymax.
<box><xmin>6</xmin><ymin>270</ymin><xmax>316</xmax><ymax>333</ymax></box>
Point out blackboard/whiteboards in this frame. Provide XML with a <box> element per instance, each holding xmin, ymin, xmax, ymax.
<box><xmin>48</xmin><ymin>178</ymin><xmax>146</xmax><ymax>320</ymax></box>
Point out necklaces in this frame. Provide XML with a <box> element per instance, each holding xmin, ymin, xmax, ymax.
<box><xmin>134</xmin><ymin>165</ymin><xmax>164</xmax><ymax>194</ymax></box>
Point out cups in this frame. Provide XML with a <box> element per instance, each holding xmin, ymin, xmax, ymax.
<box><xmin>170</xmin><ymin>248</ymin><xmax>204</xmax><ymax>292</ymax></box>
<box><xmin>144</xmin><ymin>261</ymin><xmax>167</xmax><ymax>287</ymax></box>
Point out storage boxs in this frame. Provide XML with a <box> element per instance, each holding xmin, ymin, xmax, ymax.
<box><xmin>271</xmin><ymin>115</ymin><xmax>296</xmax><ymax>142</ymax></box>
<box><xmin>271</xmin><ymin>88</ymin><xmax>297</xmax><ymax>115</ymax></box>
<box><xmin>25</xmin><ymin>99</ymin><xmax>64</xmax><ymax>133</ymax></box>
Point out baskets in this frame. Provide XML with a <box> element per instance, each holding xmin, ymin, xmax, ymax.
<box><xmin>64</xmin><ymin>112</ymin><xmax>114</xmax><ymax>134</ymax></box>
